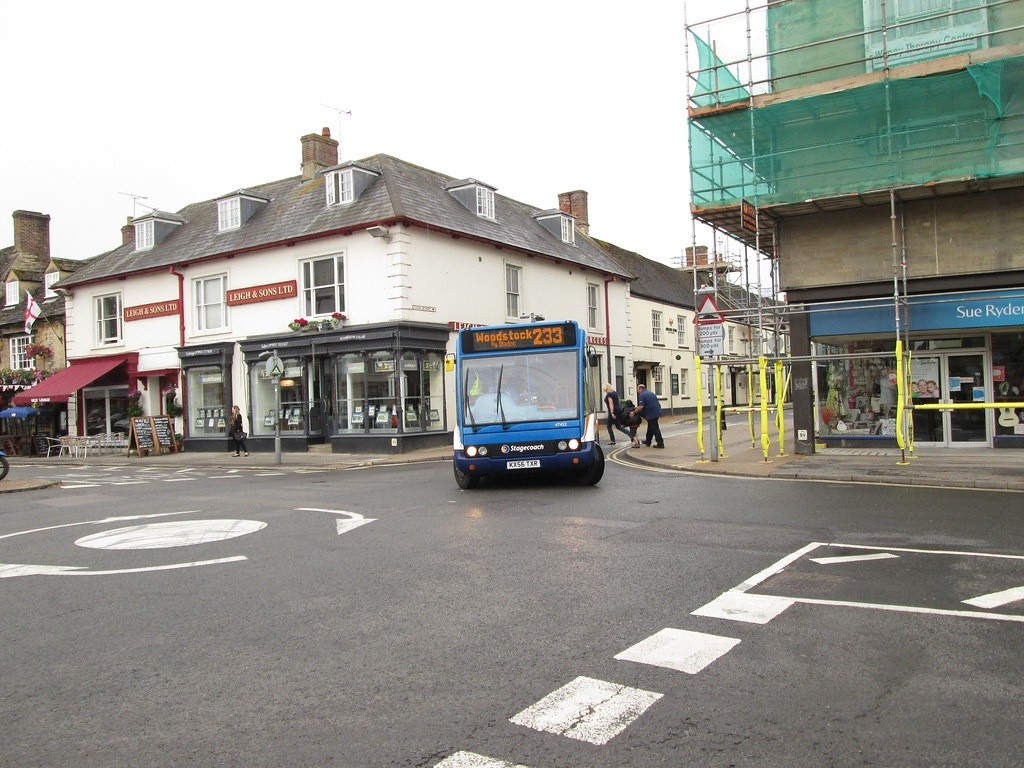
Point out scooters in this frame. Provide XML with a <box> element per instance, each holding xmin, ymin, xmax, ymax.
<box><xmin>0</xmin><ymin>451</ymin><xmax>9</xmax><ymax>480</ymax></box>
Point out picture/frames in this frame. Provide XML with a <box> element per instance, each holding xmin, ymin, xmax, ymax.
<box><xmin>405</xmin><ymin>410</ymin><xmax>418</xmax><ymax>423</ymax></box>
<box><xmin>193</xmin><ymin>407</ymin><xmax>226</xmax><ymax>429</ymax></box>
<box><xmin>288</xmin><ymin>415</ymin><xmax>300</xmax><ymax>426</ymax></box>
<box><xmin>292</xmin><ymin>407</ymin><xmax>303</xmax><ymax>415</ymax></box>
<box><xmin>374</xmin><ymin>411</ymin><xmax>390</xmax><ymax>424</ymax></box>
<box><xmin>264</xmin><ymin>415</ymin><xmax>275</xmax><ymax>426</ymax></box>
<box><xmin>351</xmin><ymin>412</ymin><xmax>363</xmax><ymax>424</ymax></box>
<box><xmin>285</xmin><ymin>409</ymin><xmax>292</xmax><ymax>419</ymax></box>
<box><xmin>425</xmin><ymin>409</ymin><xmax>440</xmax><ymax>421</ymax></box>
<box><xmin>269</xmin><ymin>409</ymin><xmax>276</xmax><ymax>417</ymax></box>
<box><xmin>278</xmin><ymin>409</ymin><xmax>284</xmax><ymax>420</ymax></box>
<box><xmin>354</xmin><ymin>404</ymin><xmax>388</xmax><ymax>416</ymax></box>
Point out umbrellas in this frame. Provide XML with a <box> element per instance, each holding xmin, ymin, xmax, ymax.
<box><xmin>0</xmin><ymin>406</ymin><xmax>39</xmax><ymax>458</ymax></box>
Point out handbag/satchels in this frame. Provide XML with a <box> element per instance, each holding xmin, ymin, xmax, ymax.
<box><xmin>234</xmin><ymin>432</ymin><xmax>247</xmax><ymax>441</ymax></box>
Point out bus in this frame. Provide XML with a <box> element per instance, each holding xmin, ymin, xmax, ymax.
<box><xmin>443</xmin><ymin>320</ymin><xmax>606</xmax><ymax>490</ymax></box>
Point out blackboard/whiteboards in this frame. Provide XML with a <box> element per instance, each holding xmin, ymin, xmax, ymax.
<box><xmin>29</xmin><ymin>432</ymin><xmax>55</xmax><ymax>458</ymax></box>
<box><xmin>127</xmin><ymin>417</ymin><xmax>159</xmax><ymax>451</ymax></box>
<box><xmin>151</xmin><ymin>415</ymin><xmax>178</xmax><ymax>448</ymax></box>
<box><xmin>53</xmin><ymin>433</ymin><xmax>69</xmax><ymax>456</ymax></box>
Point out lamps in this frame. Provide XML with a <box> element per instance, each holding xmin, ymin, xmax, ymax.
<box><xmin>366</xmin><ymin>225</ymin><xmax>392</xmax><ymax>243</ymax></box>
<box><xmin>53</xmin><ymin>288</ymin><xmax>74</xmax><ymax>297</ymax></box>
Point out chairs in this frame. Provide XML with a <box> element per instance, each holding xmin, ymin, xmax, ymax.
<box><xmin>45</xmin><ymin>432</ymin><xmax>127</xmax><ymax>458</ymax></box>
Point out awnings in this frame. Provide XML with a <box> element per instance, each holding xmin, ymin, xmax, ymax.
<box><xmin>13</xmin><ymin>360</ymin><xmax>127</xmax><ymax>404</ymax></box>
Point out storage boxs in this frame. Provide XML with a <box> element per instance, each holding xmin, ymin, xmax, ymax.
<box><xmin>855</xmin><ymin>396</ymin><xmax>869</xmax><ymax>412</ymax></box>
<box><xmin>878</xmin><ymin>418</ymin><xmax>896</xmax><ymax>435</ymax></box>
<box><xmin>871</xmin><ymin>397</ymin><xmax>881</xmax><ymax>413</ymax></box>
<box><xmin>842</xmin><ymin>408</ymin><xmax>860</xmax><ymax>422</ymax></box>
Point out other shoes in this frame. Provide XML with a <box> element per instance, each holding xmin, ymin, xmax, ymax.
<box><xmin>232</xmin><ymin>454</ymin><xmax>240</xmax><ymax>457</ymax></box>
<box><xmin>653</xmin><ymin>444</ymin><xmax>664</xmax><ymax>448</ymax></box>
<box><xmin>631</xmin><ymin>436</ymin><xmax>633</xmax><ymax>442</ymax></box>
<box><xmin>606</xmin><ymin>441</ymin><xmax>615</xmax><ymax>445</ymax></box>
<box><xmin>245</xmin><ymin>451</ymin><xmax>249</xmax><ymax>456</ymax></box>
<box><xmin>632</xmin><ymin>441</ymin><xmax>640</xmax><ymax>448</ymax></box>
<box><xmin>642</xmin><ymin>440</ymin><xmax>650</xmax><ymax>446</ymax></box>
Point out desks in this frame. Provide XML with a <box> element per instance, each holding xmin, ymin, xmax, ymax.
<box><xmin>0</xmin><ymin>435</ymin><xmax>28</xmax><ymax>457</ymax></box>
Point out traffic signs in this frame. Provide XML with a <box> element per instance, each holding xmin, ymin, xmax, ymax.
<box><xmin>699</xmin><ymin>336</ymin><xmax>724</xmax><ymax>356</ymax></box>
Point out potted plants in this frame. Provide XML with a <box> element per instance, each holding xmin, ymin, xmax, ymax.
<box><xmin>322</xmin><ymin>319</ymin><xmax>331</xmax><ymax>330</ymax></box>
<box><xmin>0</xmin><ymin>368</ymin><xmax>49</xmax><ymax>384</ymax></box>
<box><xmin>308</xmin><ymin>320</ymin><xmax>319</xmax><ymax>332</ymax></box>
<box><xmin>169</xmin><ymin>433</ymin><xmax>182</xmax><ymax>453</ymax></box>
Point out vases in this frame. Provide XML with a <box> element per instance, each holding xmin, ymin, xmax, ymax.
<box><xmin>333</xmin><ymin>319</ymin><xmax>343</xmax><ymax>330</ymax></box>
<box><xmin>35</xmin><ymin>354</ymin><xmax>45</xmax><ymax>359</ymax></box>
<box><xmin>302</xmin><ymin>325</ymin><xmax>308</xmax><ymax>331</ymax></box>
<box><xmin>131</xmin><ymin>398</ymin><xmax>139</xmax><ymax>402</ymax></box>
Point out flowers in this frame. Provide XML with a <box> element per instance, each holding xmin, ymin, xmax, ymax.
<box><xmin>162</xmin><ymin>383</ymin><xmax>173</xmax><ymax>396</ymax></box>
<box><xmin>330</xmin><ymin>313</ymin><xmax>346</xmax><ymax>325</ymax></box>
<box><xmin>24</xmin><ymin>341</ymin><xmax>53</xmax><ymax>360</ymax></box>
<box><xmin>128</xmin><ymin>387</ymin><xmax>141</xmax><ymax>397</ymax></box>
<box><xmin>288</xmin><ymin>318</ymin><xmax>308</xmax><ymax>331</ymax></box>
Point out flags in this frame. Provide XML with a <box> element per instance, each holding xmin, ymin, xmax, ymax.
<box><xmin>25</xmin><ymin>292</ymin><xmax>43</xmax><ymax>334</ymax></box>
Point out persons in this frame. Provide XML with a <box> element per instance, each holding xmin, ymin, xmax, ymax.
<box><xmin>824</xmin><ymin>366</ymin><xmax>848</xmax><ymax>420</ymax></box>
<box><xmin>488</xmin><ymin>372</ymin><xmax>517</xmax><ymax>406</ymax></box>
<box><xmin>602</xmin><ymin>382</ymin><xmax>664</xmax><ymax>449</ymax></box>
<box><xmin>877</xmin><ymin>367</ymin><xmax>898</xmax><ymax>406</ymax></box>
<box><xmin>912</xmin><ymin>379</ymin><xmax>938</xmax><ymax>397</ymax></box>
<box><xmin>229</xmin><ymin>405</ymin><xmax>249</xmax><ymax>457</ymax></box>
